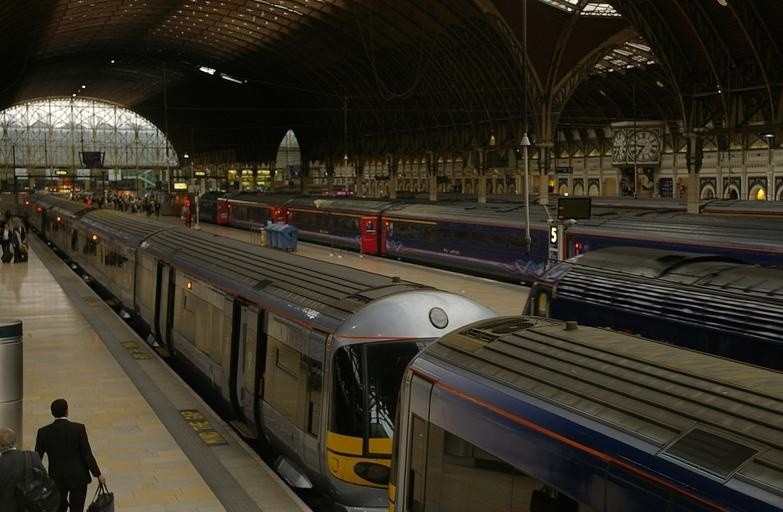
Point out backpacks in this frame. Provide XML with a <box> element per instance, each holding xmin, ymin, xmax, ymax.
<box><xmin>14</xmin><ymin>451</ymin><xmax>56</xmax><ymax>511</ymax></box>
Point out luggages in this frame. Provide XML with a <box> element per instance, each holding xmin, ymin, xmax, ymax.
<box><xmin>1</xmin><ymin>242</ymin><xmax>29</xmax><ymax>263</ymax></box>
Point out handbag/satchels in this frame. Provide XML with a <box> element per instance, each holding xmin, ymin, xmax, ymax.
<box><xmin>86</xmin><ymin>479</ymin><xmax>115</xmax><ymax>512</ymax></box>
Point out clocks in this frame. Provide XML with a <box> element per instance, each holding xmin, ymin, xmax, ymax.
<box><xmin>611</xmin><ymin>120</ymin><xmax>665</xmax><ymax>163</ymax></box>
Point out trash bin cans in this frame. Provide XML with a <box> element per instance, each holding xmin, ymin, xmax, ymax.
<box><xmin>181</xmin><ymin>205</ymin><xmax>188</xmax><ymax>220</ymax></box>
<box><xmin>264</xmin><ymin>224</ymin><xmax>299</xmax><ymax>253</ymax></box>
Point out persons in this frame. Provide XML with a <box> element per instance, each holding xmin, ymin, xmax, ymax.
<box><xmin>32</xmin><ymin>399</ymin><xmax>107</xmax><ymax>511</ymax></box>
<box><xmin>0</xmin><ymin>210</ymin><xmax>31</xmax><ymax>262</ymax></box>
<box><xmin>0</xmin><ymin>427</ymin><xmax>48</xmax><ymax>511</ymax></box>
<box><xmin>67</xmin><ymin>186</ymin><xmax>193</xmax><ymax>228</ymax></box>
